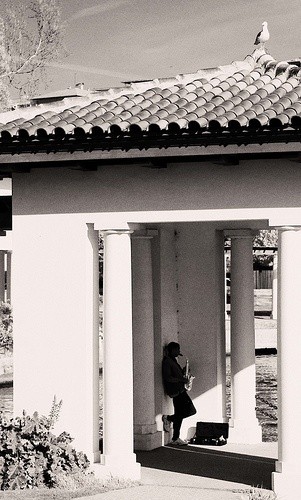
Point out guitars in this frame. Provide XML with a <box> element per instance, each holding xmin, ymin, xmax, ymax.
<box><xmin>183</xmin><ymin>359</ymin><xmax>196</xmax><ymax>391</ymax></box>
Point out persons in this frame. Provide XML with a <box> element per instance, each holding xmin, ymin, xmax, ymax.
<box><xmin>161</xmin><ymin>342</ymin><xmax>197</xmax><ymax>446</ymax></box>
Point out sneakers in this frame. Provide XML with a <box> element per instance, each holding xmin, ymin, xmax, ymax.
<box><xmin>172</xmin><ymin>438</ymin><xmax>187</xmax><ymax>445</ymax></box>
<box><xmin>162</xmin><ymin>415</ymin><xmax>171</xmax><ymax>432</ymax></box>
